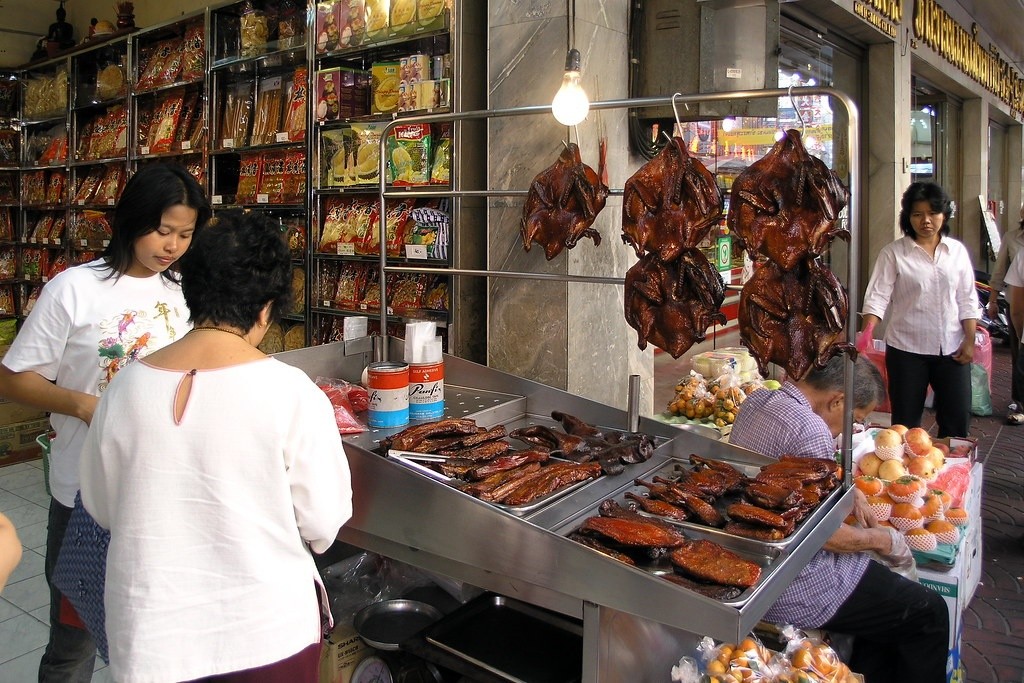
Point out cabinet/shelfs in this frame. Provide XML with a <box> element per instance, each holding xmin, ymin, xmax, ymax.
<box><xmin>0</xmin><ymin>2</ymin><xmax>486</xmax><ymax>365</ymax></box>
<box><xmin>267</xmin><ymin>334</ymin><xmax>856</xmax><ymax>683</ymax></box>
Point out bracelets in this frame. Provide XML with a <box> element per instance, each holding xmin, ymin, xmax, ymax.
<box><xmin>988</xmin><ymin>298</ymin><xmax>996</xmax><ymax>304</ymax></box>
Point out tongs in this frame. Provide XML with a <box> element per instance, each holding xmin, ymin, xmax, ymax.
<box><xmin>385</xmin><ymin>449</ymin><xmax>476</xmax><ymax>486</ymax></box>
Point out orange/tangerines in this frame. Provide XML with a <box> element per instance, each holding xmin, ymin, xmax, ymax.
<box><xmin>702</xmin><ymin>639</ymin><xmax>859</xmax><ymax>683</ymax></box>
<box><xmin>671</xmin><ymin>376</ymin><xmax>763</xmax><ymax>428</ymax></box>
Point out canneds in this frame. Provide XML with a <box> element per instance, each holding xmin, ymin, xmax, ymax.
<box><xmin>398</xmin><ymin>55</ymin><xmax>429</xmax><ymax>111</ymax></box>
<box><xmin>367</xmin><ymin>360</ymin><xmax>445</xmax><ymax>428</ymax></box>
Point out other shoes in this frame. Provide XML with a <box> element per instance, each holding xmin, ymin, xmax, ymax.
<box><xmin>1005</xmin><ymin>401</ymin><xmax>1024</xmax><ymax>427</ymax></box>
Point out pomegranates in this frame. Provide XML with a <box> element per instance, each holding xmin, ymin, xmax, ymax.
<box><xmin>860</xmin><ymin>425</ymin><xmax>946</xmax><ymax>486</ymax></box>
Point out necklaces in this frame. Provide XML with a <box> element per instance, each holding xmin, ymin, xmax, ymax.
<box><xmin>191</xmin><ymin>326</ymin><xmax>245</xmax><ymax>339</ymax></box>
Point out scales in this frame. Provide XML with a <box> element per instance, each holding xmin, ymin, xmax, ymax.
<box><xmin>350</xmin><ymin>599</ymin><xmax>444</xmax><ymax>683</ymax></box>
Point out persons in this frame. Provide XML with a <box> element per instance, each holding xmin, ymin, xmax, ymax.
<box><xmin>80</xmin><ymin>212</ymin><xmax>353</xmax><ymax>683</ymax></box>
<box><xmin>728</xmin><ymin>351</ymin><xmax>950</xmax><ymax>683</ymax></box>
<box><xmin>854</xmin><ymin>180</ymin><xmax>982</xmax><ymax>439</ymax></box>
<box><xmin>0</xmin><ymin>161</ymin><xmax>212</xmax><ymax>682</ymax></box>
<box><xmin>988</xmin><ymin>204</ymin><xmax>1024</xmax><ymax>424</ymax></box>
<box><xmin>1004</xmin><ymin>246</ymin><xmax>1024</xmax><ymax>375</ymax></box>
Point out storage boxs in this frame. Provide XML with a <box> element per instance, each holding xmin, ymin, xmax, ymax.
<box><xmin>0</xmin><ymin>394</ymin><xmax>57</xmax><ymax>497</ymax></box>
<box><xmin>320</xmin><ymin>552</ymin><xmax>449</xmax><ymax>683</ymax></box>
<box><xmin>852</xmin><ymin>429</ymin><xmax>984</xmax><ymax>683</ymax></box>
